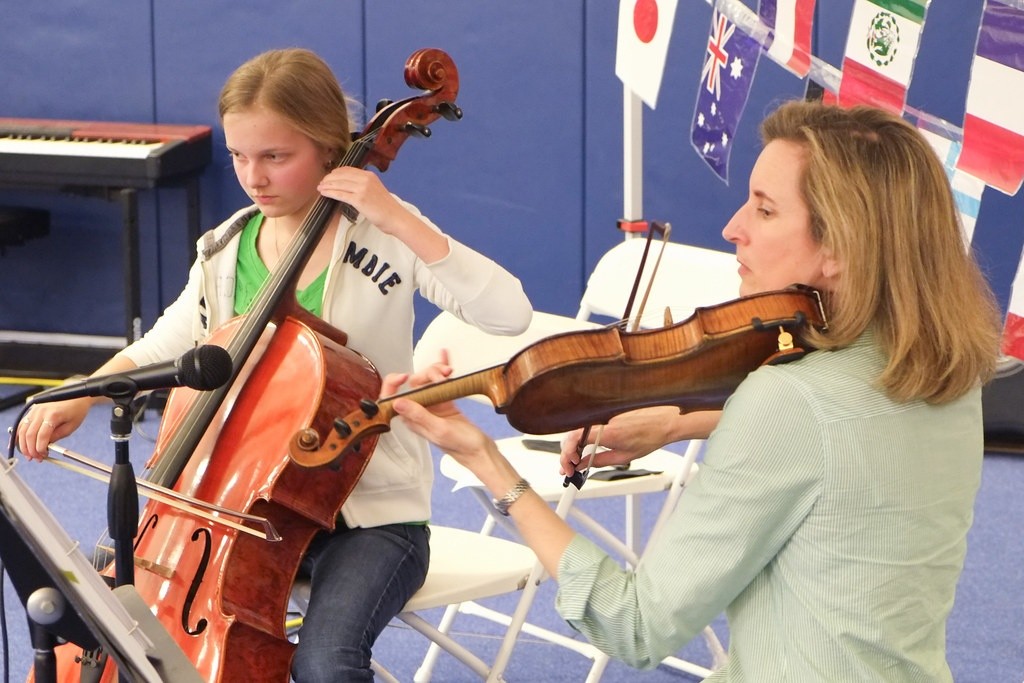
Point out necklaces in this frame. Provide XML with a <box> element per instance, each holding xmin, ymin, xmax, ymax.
<box><xmin>274</xmin><ymin>218</ymin><xmax>280</xmax><ymax>261</ymax></box>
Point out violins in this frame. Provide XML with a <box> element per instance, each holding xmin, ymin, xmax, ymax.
<box><xmin>286</xmin><ymin>282</ymin><xmax>828</xmax><ymax>472</ymax></box>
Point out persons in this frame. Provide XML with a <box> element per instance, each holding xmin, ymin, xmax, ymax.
<box><xmin>376</xmin><ymin>100</ymin><xmax>1003</xmax><ymax>681</ymax></box>
<box><xmin>11</xmin><ymin>45</ymin><xmax>534</xmax><ymax>682</ymax></box>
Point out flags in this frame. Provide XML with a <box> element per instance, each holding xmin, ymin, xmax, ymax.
<box><xmin>613</xmin><ymin>1</ymin><xmax>1024</xmax><ymax>364</ymax></box>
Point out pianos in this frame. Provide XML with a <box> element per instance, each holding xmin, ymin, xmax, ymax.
<box><xmin>0</xmin><ymin>117</ymin><xmax>216</xmax><ymax>423</ymax></box>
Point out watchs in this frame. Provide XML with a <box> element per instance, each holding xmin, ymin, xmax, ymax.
<box><xmin>491</xmin><ymin>478</ymin><xmax>530</xmax><ymax>517</ymax></box>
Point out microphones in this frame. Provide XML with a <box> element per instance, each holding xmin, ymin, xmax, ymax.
<box><xmin>33</xmin><ymin>344</ymin><xmax>233</xmax><ymax>404</ymax></box>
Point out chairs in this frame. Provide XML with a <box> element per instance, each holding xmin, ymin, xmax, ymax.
<box><xmin>409</xmin><ymin>236</ymin><xmax>728</xmax><ymax>683</ymax></box>
<box><xmin>289</xmin><ymin>310</ymin><xmax>607</xmax><ymax>683</ymax></box>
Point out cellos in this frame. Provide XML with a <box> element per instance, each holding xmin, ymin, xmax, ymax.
<box><xmin>24</xmin><ymin>48</ymin><xmax>465</xmax><ymax>683</ymax></box>
